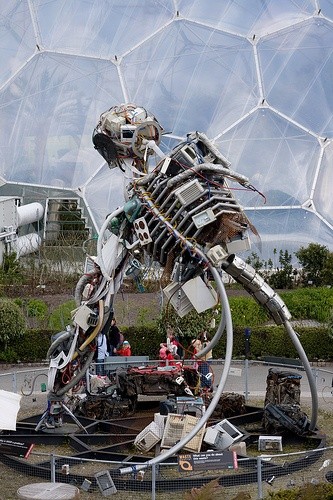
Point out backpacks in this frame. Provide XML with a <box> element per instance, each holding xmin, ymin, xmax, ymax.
<box><xmin>118</xmin><ymin>332</ymin><xmax>124</xmax><ymax>346</ymax></box>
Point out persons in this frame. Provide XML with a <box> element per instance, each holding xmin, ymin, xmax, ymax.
<box><xmin>96</xmin><ymin>331</ymin><xmax>107</xmax><ymax>376</ymax></box>
<box><xmin>107</xmin><ymin>317</ymin><xmax>120</xmax><ymax>357</ymax></box>
<box><xmin>114</xmin><ymin>340</ymin><xmax>131</xmax><ymax>356</ymax></box>
<box><xmin>160</xmin><ymin>331</ymin><xmax>213</xmax><ymax>389</ymax></box>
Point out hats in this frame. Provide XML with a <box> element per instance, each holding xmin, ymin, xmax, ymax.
<box><xmin>122</xmin><ymin>341</ymin><xmax>128</xmax><ymax>344</ymax></box>
